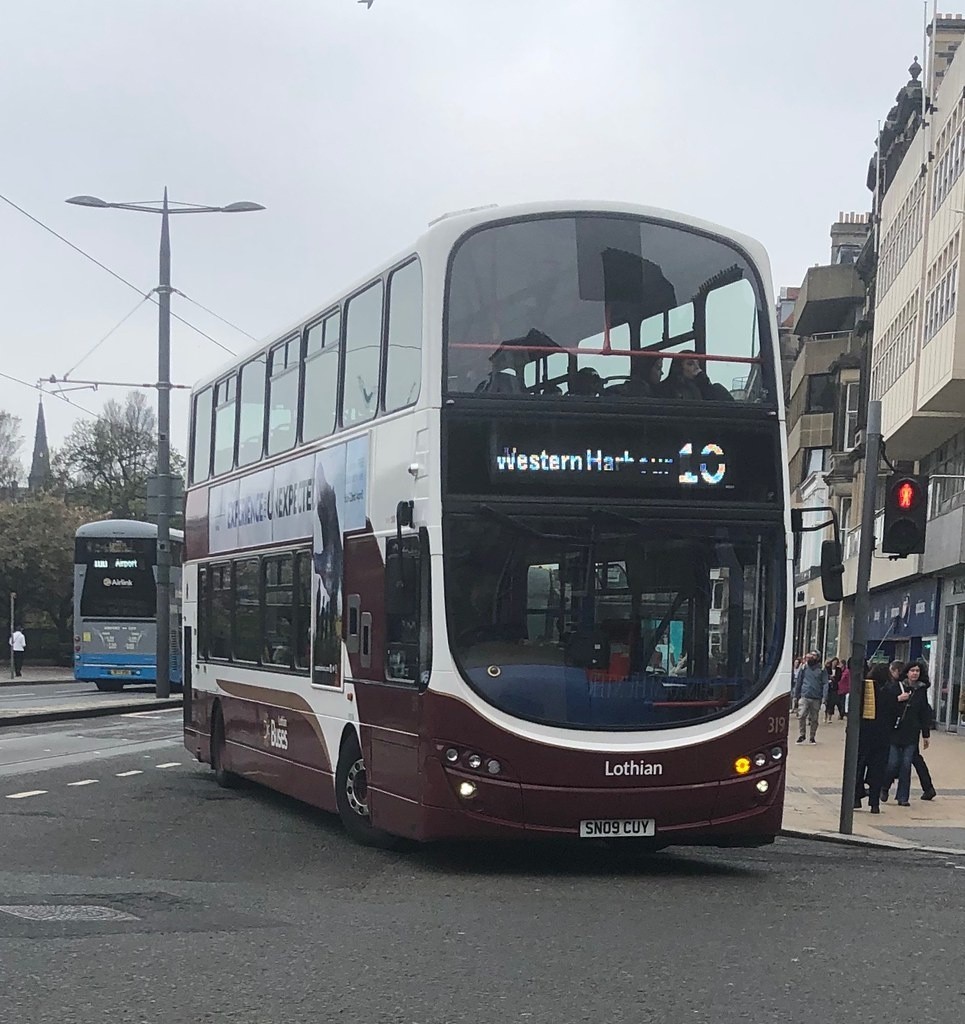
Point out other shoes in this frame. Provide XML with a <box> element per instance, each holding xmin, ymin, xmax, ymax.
<box><xmin>921</xmin><ymin>789</ymin><xmax>936</xmax><ymax>799</ymax></box>
<box><xmin>880</xmin><ymin>790</ymin><xmax>889</xmax><ymax>801</ymax></box>
<box><xmin>822</xmin><ymin>720</ymin><xmax>833</xmax><ymax>723</ymax></box>
<box><xmin>899</xmin><ymin>801</ymin><xmax>910</xmax><ymax>806</ymax></box>
<box><xmin>797</xmin><ymin>736</ymin><xmax>817</xmax><ymax>745</ymax></box>
<box><xmin>854</xmin><ymin>799</ymin><xmax>880</xmax><ymax>814</ymax></box>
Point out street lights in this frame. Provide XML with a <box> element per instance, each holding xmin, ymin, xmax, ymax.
<box><xmin>66</xmin><ymin>193</ymin><xmax>270</xmax><ymax>703</ymax></box>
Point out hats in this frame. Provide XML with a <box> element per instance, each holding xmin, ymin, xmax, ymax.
<box><xmin>813</xmin><ymin>649</ymin><xmax>821</xmax><ymax>657</ymax></box>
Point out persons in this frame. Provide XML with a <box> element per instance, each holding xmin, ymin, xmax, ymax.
<box><xmin>450</xmin><ymin>573</ymin><xmax>524</xmax><ymax>663</ymax></box>
<box><xmin>881</xmin><ymin>662</ymin><xmax>933</xmax><ymax>807</ymax></box>
<box><xmin>540</xmin><ymin>385</ymin><xmax>561</xmax><ymax>394</ymax></box>
<box><xmin>605</xmin><ymin>349</ymin><xmax>667</xmax><ymax>401</ymax></box>
<box><xmin>792</xmin><ymin>649</ymin><xmax>854</xmax><ymax>741</ymax></box>
<box><xmin>852</xmin><ymin>663</ymin><xmax>895</xmax><ymax>814</ymax></box>
<box><xmin>659</xmin><ymin>347</ymin><xmax>738</xmax><ymax>404</ymax></box>
<box><xmin>566</xmin><ymin>366</ymin><xmax>603</xmax><ymax>399</ymax></box>
<box><xmin>870</xmin><ymin>658</ymin><xmax>939</xmax><ymax>803</ymax></box>
<box><xmin>8</xmin><ymin>624</ymin><xmax>29</xmax><ymax>679</ymax></box>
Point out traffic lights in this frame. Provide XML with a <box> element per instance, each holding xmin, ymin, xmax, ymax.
<box><xmin>882</xmin><ymin>471</ymin><xmax>930</xmax><ymax>555</ymax></box>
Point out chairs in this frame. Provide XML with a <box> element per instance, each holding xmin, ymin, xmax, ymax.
<box><xmin>445</xmin><ymin>371</ymin><xmax>669</xmax><ymax>402</ymax></box>
<box><xmin>213</xmin><ymin>410</ymin><xmax>351</xmax><ymax>476</ymax></box>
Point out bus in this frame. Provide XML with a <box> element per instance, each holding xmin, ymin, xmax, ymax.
<box><xmin>176</xmin><ymin>196</ymin><xmax>849</xmax><ymax>859</ymax></box>
<box><xmin>71</xmin><ymin>518</ymin><xmax>187</xmax><ymax>690</ymax></box>
